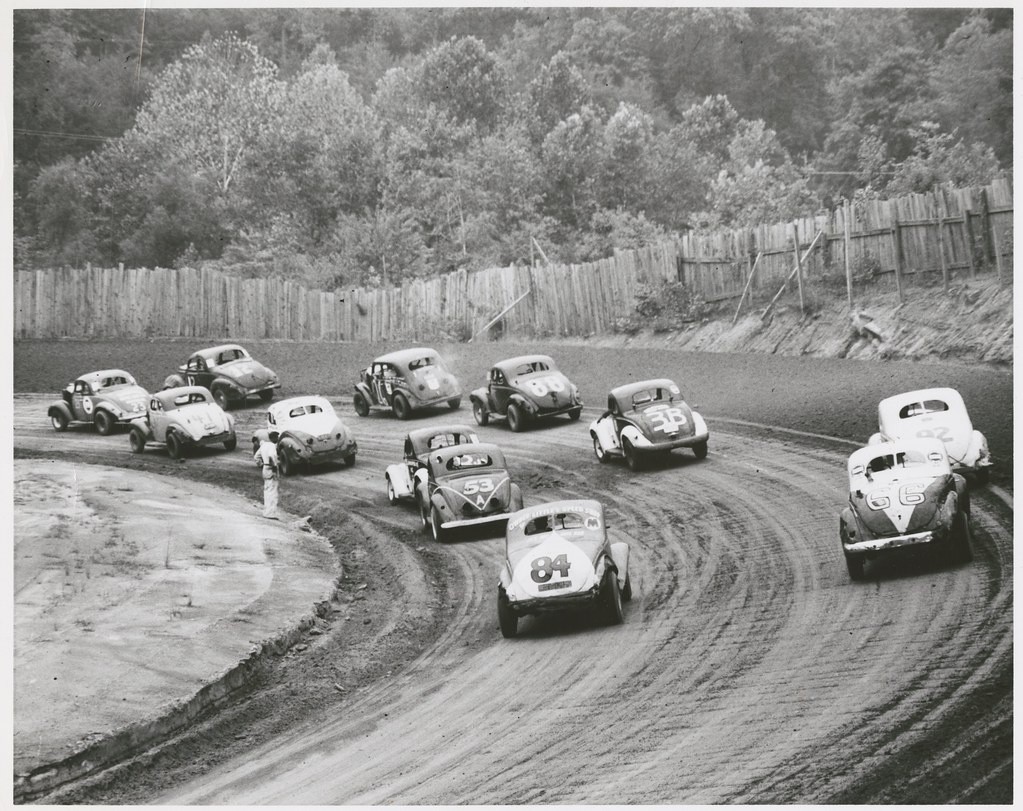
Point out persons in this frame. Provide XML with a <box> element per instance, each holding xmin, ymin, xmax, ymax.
<box><xmin>253</xmin><ymin>431</ymin><xmax>280</xmax><ymax>520</ymax></box>
<box><xmin>527</xmin><ymin>517</ymin><xmax>549</xmax><ymax>535</ymax></box>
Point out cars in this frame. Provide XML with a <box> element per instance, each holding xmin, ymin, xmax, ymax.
<box><xmin>127</xmin><ymin>381</ymin><xmax>241</xmax><ymax>461</ymax></box>
<box><xmin>380</xmin><ymin>426</ymin><xmax>479</xmax><ymax>505</ymax></box>
<box><xmin>412</xmin><ymin>441</ymin><xmax>523</xmax><ymax>541</ymax></box>
<box><xmin>49</xmin><ymin>368</ymin><xmax>157</xmax><ymax>437</ymax></box>
<box><xmin>351</xmin><ymin>346</ymin><xmax>466</xmax><ymax>419</ymax></box>
<box><xmin>833</xmin><ymin>439</ymin><xmax>973</xmax><ymax>576</ymax></box>
<box><xmin>154</xmin><ymin>343</ymin><xmax>284</xmax><ymax>409</ymax></box>
<box><xmin>495</xmin><ymin>499</ymin><xmax>634</xmax><ymax>639</ymax></box>
<box><xmin>467</xmin><ymin>350</ymin><xmax>586</xmax><ymax>427</ymax></box>
<box><xmin>588</xmin><ymin>378</ymin><xmax>712</xmax><ymax>472</ymax></box>
<box><xmin>251</xmin><ymin>393</ymin><xmax>360</xmax><ymax>476</ymax></box>
<box><xmin>867</xmin><ymin>387</ymin><xmax>993</xmax><ymax>493</ymax></box>
